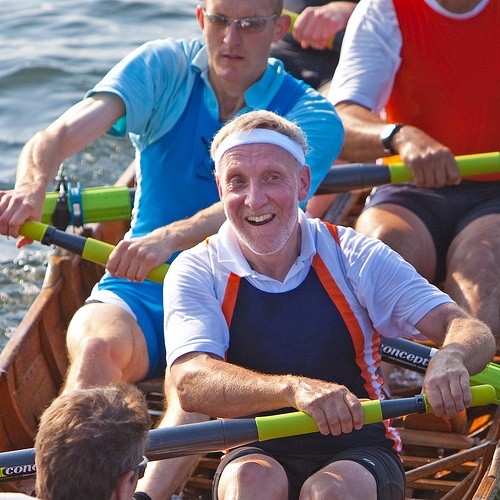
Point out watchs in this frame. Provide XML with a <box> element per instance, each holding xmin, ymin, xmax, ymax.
<box><xmin>380</xmin><ymin>120</ymin><xmax>405</xmax><ymax>155</ymax></box>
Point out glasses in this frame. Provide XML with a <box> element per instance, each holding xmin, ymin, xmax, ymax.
<box><xmin>120</xmin><ymin>455</ymin><xmax>148</xmax><ymax>480</ymax></box>
<box><xmin>201</xmin><ymin>7</ymin><xmax>281</xmax><ymax>34</ymax></box>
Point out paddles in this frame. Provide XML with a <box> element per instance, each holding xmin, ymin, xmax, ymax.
<box><xmin>12</xmin><ymin>219</ymin><xmax>500</xmax><ymax>399</ymax></box>
<box><xmin>36</xmin><ymin>152</ymin><xmax>499</xmax><ymax>226</ymax></box>
<box><xmin>0</xmin><ymin>384</ymin><xmax>497</xmax><ymax>481</ymax></box>
<box><xmin>282</xmin><ymin>8</ymin><xmax>342</xmax><ymax>51</ymax></box>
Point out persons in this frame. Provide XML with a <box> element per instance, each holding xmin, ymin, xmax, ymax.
<box><xmin>161</xmin><ymin>110</ymin><xmax>496</xmax><ymax>500</ymax></box>
<box><xmin>269</xmin><ymin>0</ymin><xmax>360</xmax><ymax>90</ymax></box>
<box><xmin>326</xmin><ymin>0</ymin><xmax>500</xmax><ymax>352</ymax></box>
<box><xmin>0</xmin><ymin>0</ymin><xmax>346</xmax><ymax>500</ymax></box>
<box><xmin>34</xmin><ymin>384</ymin><xmax>151</xmax><ymax>500</ymax></box>
